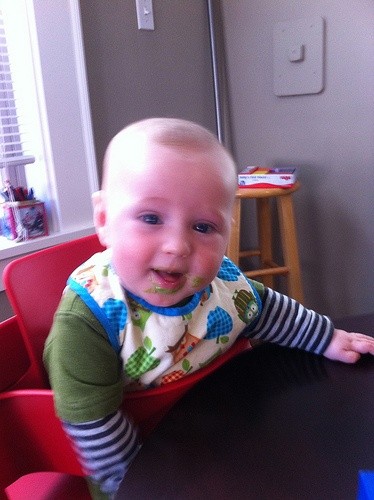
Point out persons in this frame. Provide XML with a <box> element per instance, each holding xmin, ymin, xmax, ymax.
<box><xmin>44</xmin><ymin>118</ymin><xmax>373</xmax><ymax>499</ymax></box>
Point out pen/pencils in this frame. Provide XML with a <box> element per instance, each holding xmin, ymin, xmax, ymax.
<box><xmin>0</xmin><ymin>178</ymin><xmax>35</xmax><ymax>202</ymax></box>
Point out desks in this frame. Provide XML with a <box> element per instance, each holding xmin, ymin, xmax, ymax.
<box><xmin>115</xmin><ymin>314</ymin><xmax>374</xmax><ymax>499</ymax></box>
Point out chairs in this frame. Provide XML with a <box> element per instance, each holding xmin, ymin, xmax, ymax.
<box><xmin>0</xmin><ymin>234</ymin><xmax>253</xmax><ymax>500</ymax></box>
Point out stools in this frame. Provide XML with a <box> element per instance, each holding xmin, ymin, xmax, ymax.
<box><xmin>224</xmin><ymin>180</ymin><xmax>305</xmax><ymax>307</ymax></box>
